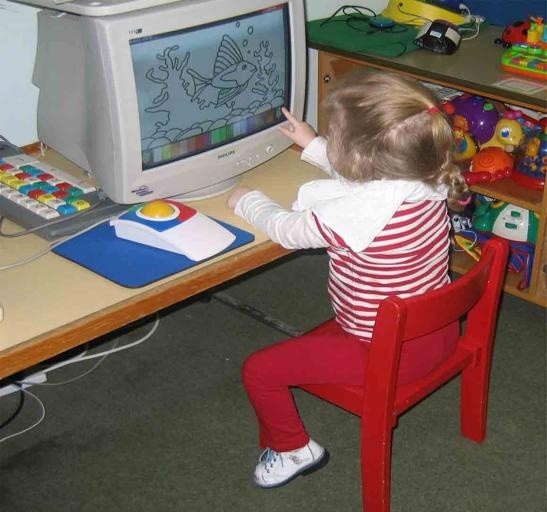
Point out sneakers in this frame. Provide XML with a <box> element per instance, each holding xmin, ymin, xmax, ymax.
<box><xmin>253</xmin><ymin>437</ymin><xmax>329</xmax><ymax>489</ymax></box>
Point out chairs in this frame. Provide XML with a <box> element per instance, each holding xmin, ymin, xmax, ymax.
<box><xmin>290</xmin><ymin>238</ymin><xmax>511</xmax><ymax>512</ymax></box>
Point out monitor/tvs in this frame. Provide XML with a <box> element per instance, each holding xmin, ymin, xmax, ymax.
<box><xmin>36</xmin><ymin>0</ymin><xmax>308</xmax><ymax>205</ymax></box>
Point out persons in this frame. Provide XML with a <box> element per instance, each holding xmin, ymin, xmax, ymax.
<box><xmin>225</xmin><ymin>71</ymin><xmax>472</xmax><ymax>490</ymax></box>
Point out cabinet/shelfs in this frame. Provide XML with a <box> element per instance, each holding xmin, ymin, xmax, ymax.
<box><xmin>316</xmin><ymin>50</ymin><xmax>547</xmax><ymax>311</ymax></box>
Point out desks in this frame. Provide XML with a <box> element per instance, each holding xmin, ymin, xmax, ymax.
<box><xmin>0</xmin><ymin>137</ymin><xmax>339</xmax><ymax>385</ymax></box>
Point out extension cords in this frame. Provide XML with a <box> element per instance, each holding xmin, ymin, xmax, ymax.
<box><xmin>0</xmin><ymin>371</ymin><xmax>47</xmax><ymax>397</ymax></box>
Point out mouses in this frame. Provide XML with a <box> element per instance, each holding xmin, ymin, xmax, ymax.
<box><xmin>108</xmin><ymin>200</ymin><xmax>236</xmax><ymax>261</ymax></box>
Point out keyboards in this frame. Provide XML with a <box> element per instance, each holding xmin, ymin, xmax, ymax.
<box><xmin>0</xmin><ymin>153</ymin><xmax>132</xmax><ymax>241</ymax></box>
